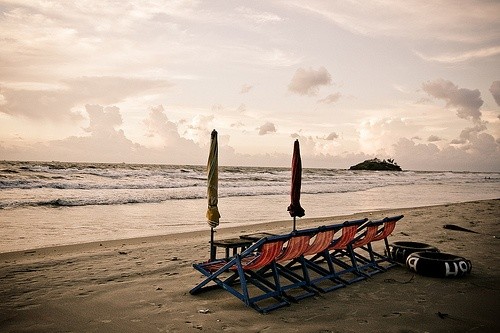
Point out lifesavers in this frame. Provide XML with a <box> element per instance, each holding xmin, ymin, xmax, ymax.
<box><xmin>406</xmin><ymin>251</ymin><xmax>472</xmax><ymax>279</ymax></box>
<box><xmin>384</xmin><ymin>240</ymin><xmax>439</xmax><ymax>263</ymax></box>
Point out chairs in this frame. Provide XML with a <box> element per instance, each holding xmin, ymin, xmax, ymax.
<box><xmin>189</xmin><ymin>213</ymin><xmax>405</xmax><ymax>315</ymax></box>
